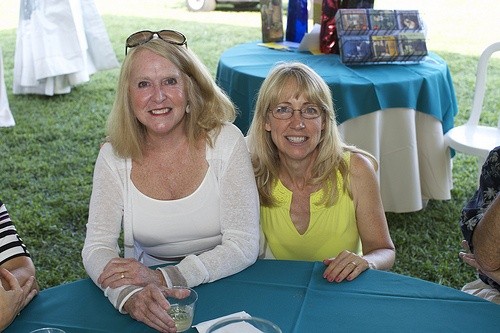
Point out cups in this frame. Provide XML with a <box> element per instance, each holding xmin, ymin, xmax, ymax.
<box><xmin>30</xmin><ymin>327</ymin><xmax>66</xmax><ymax>333</ymax></box>
<box><xmin>160</xmin><ymin>286</ymin><xmax>198</xmax><ymax>333</ymax></box>
<box><xmin>206</xmin><ymin>316</ymin><xmax>283</xmax><ymax>333</ymax></box>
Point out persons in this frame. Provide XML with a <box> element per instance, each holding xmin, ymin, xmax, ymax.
<box><xmin>0</xmin><ymin>199</ymin><xmax>38</xmax><ymax>333</ymax></box>
<box><xmin>249</xmin><ymin>63</ymin><xmax>396</xmax><ymax>283</ymax></box>
<box><xmin>460</xmin><ymin>146</ymin><xmax>500</xmax><ymax>305</ymax></box>
<box><xmin>81</xmin><ymin>29</ymin><xmax>259</xmax><ymax>333</ymax></box>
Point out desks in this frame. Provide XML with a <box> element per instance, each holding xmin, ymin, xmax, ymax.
<box><xmin>0</xmin><ymin>258</ymin><xmax>500</xmax><ymax>333</ymax></box>
<box><xmin>215</xmin><ymin>36</ymin><xmax>458</xmax><ymax>214</ymax></box>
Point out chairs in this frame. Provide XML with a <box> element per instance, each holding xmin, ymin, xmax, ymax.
<box><xmin>445</xmin><ymin>42</ymin><xmax>500</xmax><ymax>187</ymax></box>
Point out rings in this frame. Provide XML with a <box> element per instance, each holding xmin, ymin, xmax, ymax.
<box><xmin>121</xmin><ymin>273</ymin><xmax>124</xmax><ymax>279</ymax></box>
<box><xmin>351</xmin><ymin>261</ymin><xmax>356</xmax><ymax>266</ymax></box>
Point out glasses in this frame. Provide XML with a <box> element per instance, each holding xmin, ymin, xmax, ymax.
<box><xmin>268</xmin><ymin>104</ymin><xmax>326</xmax><ymax>120</ymax></box>
<box><xmin>125</xmin><ymin>29</ymin><xmax>187</xmax><ymax>57</ymax></box>
